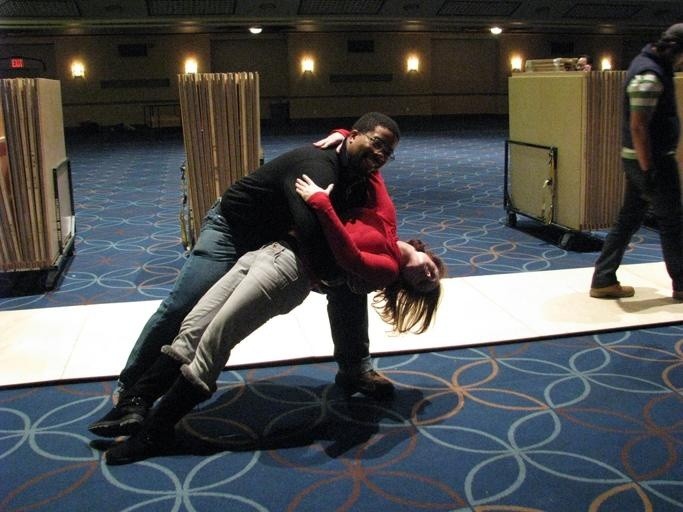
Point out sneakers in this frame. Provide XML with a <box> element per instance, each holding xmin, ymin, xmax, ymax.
<box><xmin>589</xmin><ymin>282</ymin><xmax>635</xmax><ymax>299</ymax></box>
<box><xmin>334</xmin><ymin>367</ymin><xmax>395</xmax><ymax>403</ymax></box>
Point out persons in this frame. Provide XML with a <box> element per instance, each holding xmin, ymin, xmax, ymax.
<box><xmin>112</xmin><ymin>114</ymin><xmax>400</xmax><ymax>407</ymax></box>
<box><xmin>590</xmin><ymin>23</ymin><xmax>683</xmax><ymax>299</ymax></box>
<box><xmin>86</xmin><ymin>128</ymin><xmax>445</xmax><ymax>465</ymax></box>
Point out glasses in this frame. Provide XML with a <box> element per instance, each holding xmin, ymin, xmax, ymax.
<box><xmin>359</xmin><ymin>130</ymin><xmax>396</xmax><ymax>166</ymax></box>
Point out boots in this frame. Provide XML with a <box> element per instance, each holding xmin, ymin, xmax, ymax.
<box><xmin>89</xmin><ymin>345</ymin><xmax>212</xmax><ymax>464</ymax></box>
